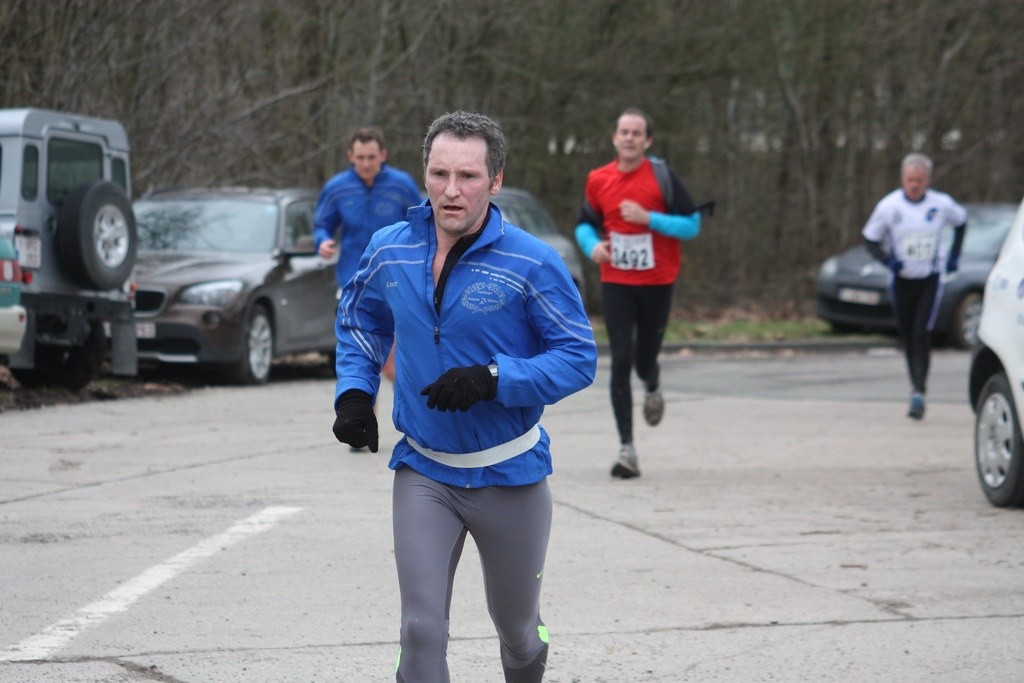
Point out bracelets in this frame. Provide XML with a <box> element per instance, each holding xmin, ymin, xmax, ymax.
<box><xmin>486</xmin><ymin>364</ymin><xmax>498</xmax><ymax>385</ymax></box>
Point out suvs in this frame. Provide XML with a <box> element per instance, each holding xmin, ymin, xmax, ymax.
<box><xmin>1</xmin><ymin>109</ymin><xmax>141</xmax><ymax>389</ymax></box>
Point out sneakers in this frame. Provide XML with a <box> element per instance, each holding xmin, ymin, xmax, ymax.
<box><xmin>908</xmin><ymin>404</ymin><xmax>924</xmax><ymax>419</ymax></box>
<box><xmin>610</xmin><ymin>449</ymin><xmax>641</xmax><ymax>478</ymax></box>
<box><xmin>643</xmin><ymin>390</ymin><xmax>664</xmax><ymax>426</ymax></box>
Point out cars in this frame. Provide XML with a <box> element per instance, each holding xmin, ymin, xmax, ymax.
<box><xmin>130</xmin><ymin>186</ymin><xmax>341</xmax><ymax>387</ymax></box>
<box><xmin>966</xmin><ymin>197</ymin><xmax>1023</xmax><ymax>507</ymax></box>
<box><xmin>414</xmin><ymin>191</ymin><xmax>585</xmax><ymax>312</ymax></box>
<box><xmin>814</xmin><ymin>200</ymin><xmax>1019</xmax><ymax>350</ymax></box>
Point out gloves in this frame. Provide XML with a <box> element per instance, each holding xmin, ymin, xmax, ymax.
<box><xmin>888</xmin><ymin>258</ymin><xmax>902</xmax><ymax>273</ymax></box>
<box><xmin>947</xmin><ymin>256</ymin><xmax>957</xmax><ymax>271</ymax></box>
<box><xmin>333</xmin><ymin>388</ymin><xmax>378</xmax><ymax>453</ymax></box>
<box><xmin>420</xmin><ymin>364</ymin><xmax>496</xmax><ymax>412</ymax></box>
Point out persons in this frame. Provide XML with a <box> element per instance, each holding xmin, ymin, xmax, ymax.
<box><xmin>571</xmin><ymin>106</ymin><xmax>715</xmax><ymax>478</ymax></box>
<box><xmin>332</xmin><ymin>110</ymin><xmax>597</xmax><ymax>683</ymax></box>
<box><xmin>312</xmin><ymin>126</ymin><xmax>421</xmax><ymax>383</ymax></box>
<box><xmin>860</xmin><ymin>153</ymin><xmax>969</xmax><ymax>420</ymax></box>
